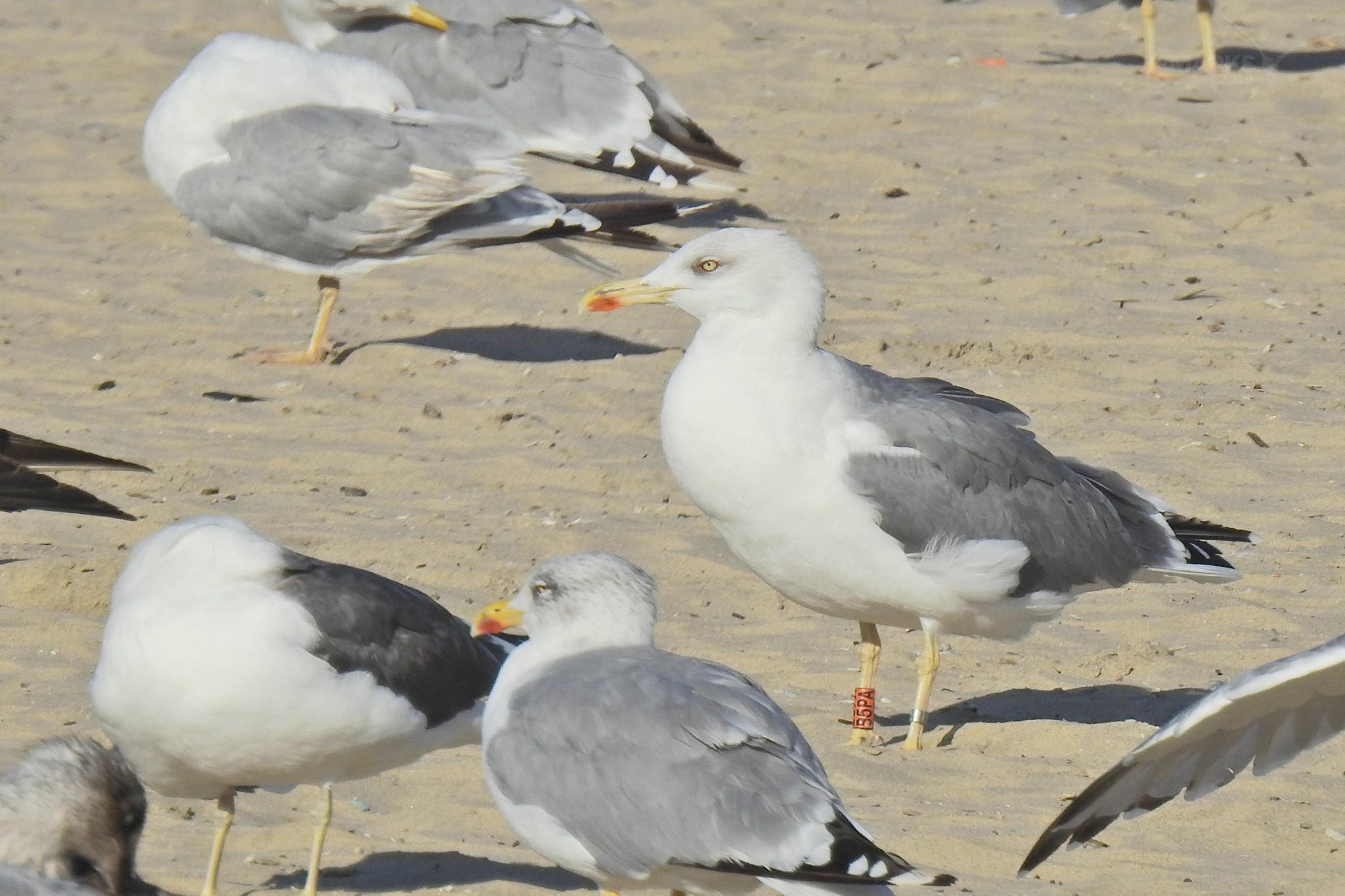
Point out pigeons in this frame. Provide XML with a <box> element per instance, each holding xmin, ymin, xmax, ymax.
<box><xmin>2</xmin><ymin>514</ymin><xmax>1343</xmax><ymax>895</ymax></box>
<box><xmin>581</xmin><ymin>227</ymin><xmax>1264</xmax><ymax>748</ymax></box>
<box><xmin>139</xmin><ymin>3</ymin><xmax>749</xmax><ymax>370</ymax></box>
